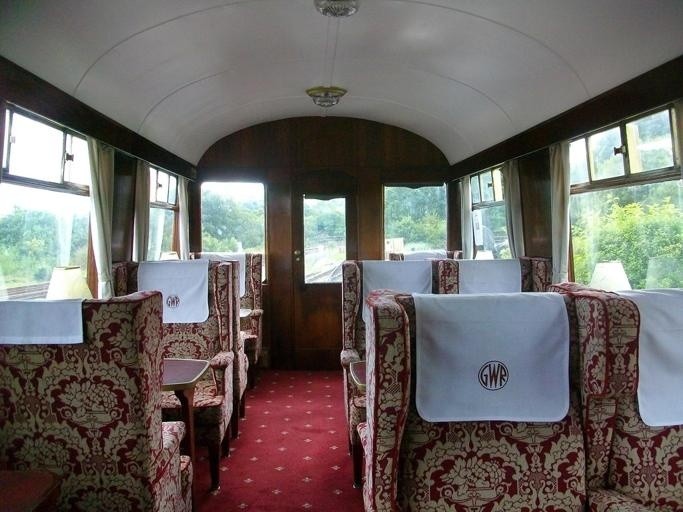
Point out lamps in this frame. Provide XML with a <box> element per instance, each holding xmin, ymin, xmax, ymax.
<box><xmin>307</xmin><ymin>0</ymin><xmax>360</xmax><ymax>118</ymax></box>
